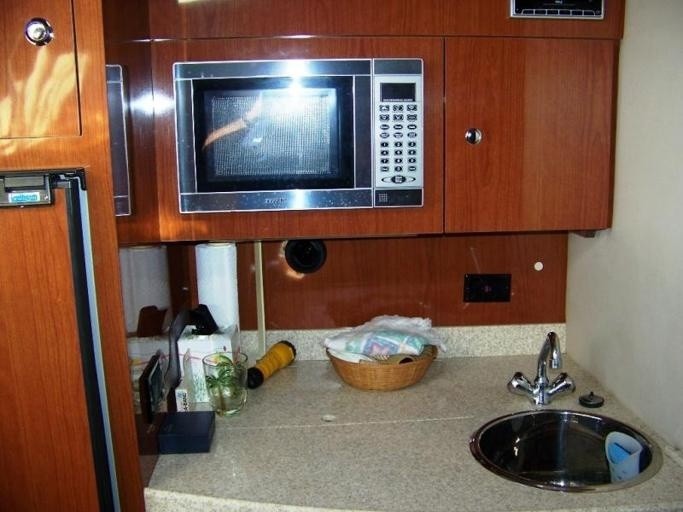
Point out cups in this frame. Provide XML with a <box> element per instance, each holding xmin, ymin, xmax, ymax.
<box><xmin>203</xmin><ymin>352</ymin><xmax>248</xmax><ymax>418</ymax></box>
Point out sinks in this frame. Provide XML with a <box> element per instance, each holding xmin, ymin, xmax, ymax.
<box><xmin>469</xmin><ymin>409</ymin><xmax>664</xmax><ymax>493</ymax></box>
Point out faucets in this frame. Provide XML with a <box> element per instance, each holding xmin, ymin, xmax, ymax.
<box><xmin>534</xmin><ymin>330</ymin><xmax>561</xmax><ymax>405</ymax></box>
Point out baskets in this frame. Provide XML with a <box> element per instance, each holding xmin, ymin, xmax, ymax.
<box><xmin>325</xmin><ymin>344</ymin><xmax>437</xmax><ymax>391</ymax></box>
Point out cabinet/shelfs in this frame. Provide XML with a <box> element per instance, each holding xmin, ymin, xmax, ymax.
<box><xmin>443</xmin><ymin>37</ymin><xmax>622</xmax><ymax>236</ymax></box>
<box><xmin>0</xmin><ymin>3</ymin><xmax>102</xmax><ymax>175</ymax></box>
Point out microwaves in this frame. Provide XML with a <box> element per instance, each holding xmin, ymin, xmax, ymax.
<box><xmin>173</xmin><ymin>58</ymin><xmax>423</xmax><ymax>214</ymax></box>
